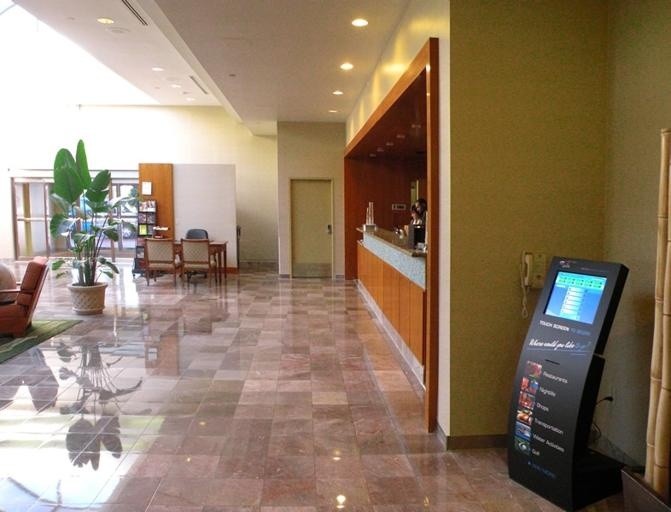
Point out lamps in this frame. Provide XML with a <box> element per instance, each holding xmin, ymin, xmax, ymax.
<box><xmin>0</xmin><ymin>255</ymin><xmax>51</xmax><ymax>338</ymax></box>
<box><xmin>144</xmin><ymin>229</ymin><xmax>229</xmax><ymax>290</ymax></box>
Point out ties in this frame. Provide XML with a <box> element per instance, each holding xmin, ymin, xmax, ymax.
<box><xmin>543</xmin><ymin>269</ymin><xmax>609</xmax><ymax>325</ymax></box>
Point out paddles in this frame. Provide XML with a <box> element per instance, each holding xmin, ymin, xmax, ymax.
<box><xmin>0</xmin><ymin>318</ymin><xmax>83</xmax><ymax>365</ymax></box>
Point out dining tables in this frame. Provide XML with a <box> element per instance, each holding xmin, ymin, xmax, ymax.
<box><xmin>46</xmin><ymin>138</ymin><xmax>145</xmax><ymax>315</ymax></box>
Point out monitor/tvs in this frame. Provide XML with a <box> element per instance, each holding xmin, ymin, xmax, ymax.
<box><xmin>136</xmin><ymin>247</ymin><xmax>145</xmax><ymax>259</ymax></box>
<box><xmin>147</xmin><ymin>200</ymin><xmax>156</xmax><ymax>213</ymax></box>
<box><xmin>147</xmin><ymin>225</ymin><xmax>154</xmax><ymax>236</ymax></box>
<box><xmin>137</xmin><ymin>236</ymin><xmax>145</xmax><ymax>246</ymax></box>
<box><xmin>135</xmin><ymin>257</ymin><xmax>146</xmax><ymax>270</ymax></box>
<box><xmin>137</xmin><ymin>200</ymin><xmax>147</xmax><ymax>212</ymax></box>
<box><xmin>137</xmin><ymin>213</ymin><xmax>146</xmax><ymax>224</ymax></box>
<box><xmin>146</xmin><ymin>213</ymin><xmax>156</xmax><ymax>225</ymax></box>
<box><xmin>138</xmin><ymin>225</ymin><xmax>147</xmax><ymax>236</ymax></box>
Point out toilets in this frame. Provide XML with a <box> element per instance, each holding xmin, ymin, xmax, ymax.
<box><xmin>521</xmin><ymin>250</ymin><xmax>547</xmax><ymax>291</ymax></box>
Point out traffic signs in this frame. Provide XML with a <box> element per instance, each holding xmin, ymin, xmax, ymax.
<box><xmin>609</xmin><ymin>387</ymin><xmax>616</xmax><ymax>405</ymax></box>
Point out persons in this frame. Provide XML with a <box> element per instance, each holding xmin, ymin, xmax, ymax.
<box><xmin>409</xmin><ymin>204</ymin><xmax>422</xmax><ymax>228</ymax></box>
<box><xmin>414</xmin><ymin>198</ymin><xmax>427</xmax><ymax>225</ymax></box>
